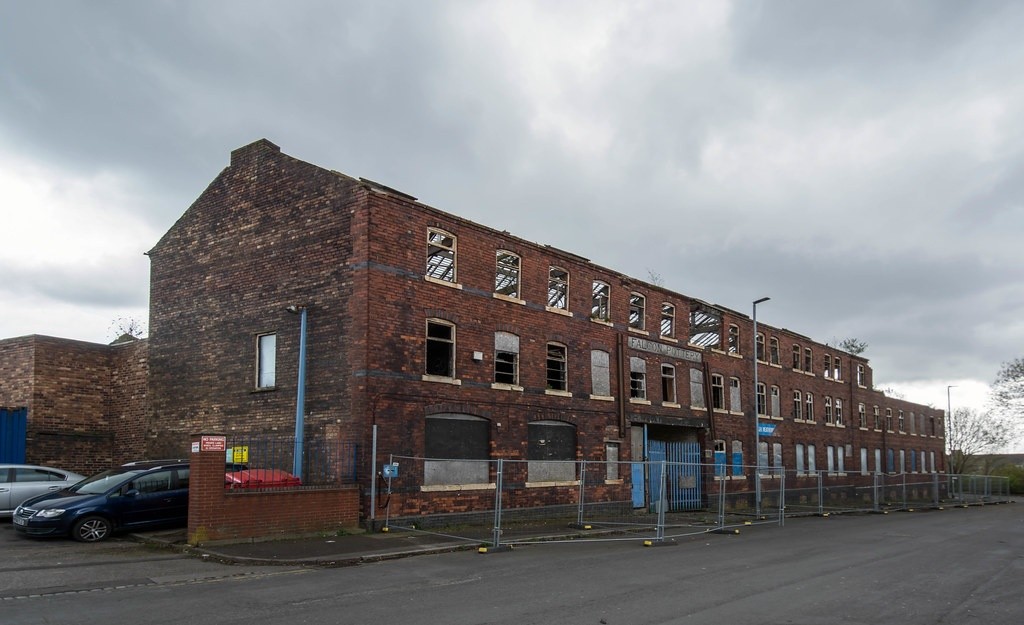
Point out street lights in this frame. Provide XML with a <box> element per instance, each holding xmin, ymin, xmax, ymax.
<box><xmin>947</xmin><ymin>385</ymin><xmax>959</xmax><ymax>496</ymax></box>
<box><xmin>753</xmin><ymin>297</ymin><xmax>771</xmax><ymax>505</ymax></box>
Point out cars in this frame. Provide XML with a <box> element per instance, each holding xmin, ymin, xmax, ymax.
<box><xmin>11</xmin><ymin>457</ymin><xmax>247</xmax><ymax>544</ymax></box>
<box><xmin>0</xmin><ymin>465</ymin><xmax>90</xmax><ymax>517</ymax></box>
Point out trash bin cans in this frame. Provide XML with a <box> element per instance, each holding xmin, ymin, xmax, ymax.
<box><xmin>225</xmin><ymin>467</ymin><xmax>303</xmax><ymax>488</ymax></box>
